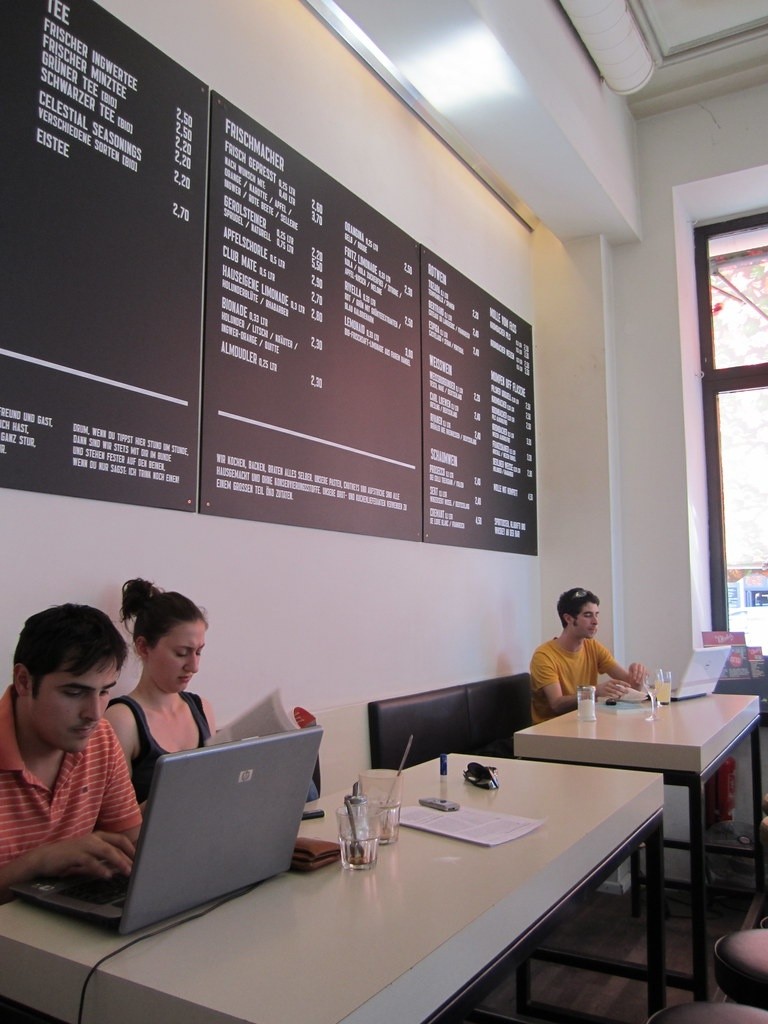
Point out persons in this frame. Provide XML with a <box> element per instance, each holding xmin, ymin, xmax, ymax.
<box><xmin>103</xmin><ymin>579</ymin><xmax>217</xmax><ymax>813</ymax></box>
<box><xmin>0</xmin><ymin>600</ymin><xmax>142</xmax><ymax>892</ymax></box>
<box><xmin>525</xmin><ymin>582</ymin><xmax>650</xmax><ymax>729</ymax></box>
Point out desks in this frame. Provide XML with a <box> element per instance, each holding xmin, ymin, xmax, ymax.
<box><xmin>513</xmin><ymin>692</ymin><xmax>768</xmax><ymax>1024</ymax></box>
<box><xmin>0</xmin><ymin>752</ymin><xmax>666</xmax><ymax>1024</ymax></box>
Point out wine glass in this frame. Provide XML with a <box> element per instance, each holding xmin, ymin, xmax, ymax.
<box><xmin>643</xmin><ymin>667</ymin><xmax>664</xmax><ymax>721</ymax></box>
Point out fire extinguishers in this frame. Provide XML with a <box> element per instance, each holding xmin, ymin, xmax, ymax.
<box><xmin>705</xmin><ymin>758</ymin><xmax>736</xmax><ymax>828</ymax></box>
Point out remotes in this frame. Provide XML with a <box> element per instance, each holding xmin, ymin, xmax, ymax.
<box><xmin>419</xmin><ymin>798</ymin><xmax>459</xmax><ymax>812</ymax></box>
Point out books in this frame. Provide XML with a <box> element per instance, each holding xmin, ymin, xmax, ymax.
<box><xmin>204</xmin><ymin>687</ymin><xmax>321</xmax><ymax>803</ymax></box>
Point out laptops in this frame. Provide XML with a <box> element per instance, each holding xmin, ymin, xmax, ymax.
<box><xmin>645</xmin><ymin>645</ymin><xmax>732</xmax><ymax>701</ymax></box>
<box><xmin>9</xmin><ymin>726</ymin><xmax>323</xmax><ymax>935</ymax></box>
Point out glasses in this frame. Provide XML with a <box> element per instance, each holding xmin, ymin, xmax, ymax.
<box><xmin>571</xmin><ymin>589</ymin><xmax>588</xmax><ymax>600</ymax></box>
<box><xmin>463</xmin><ymin>762</ymin><xmax>499</xmax><ymax>789</ymax></box>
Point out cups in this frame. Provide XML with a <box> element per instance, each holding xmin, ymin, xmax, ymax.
<box><xmin>578</xmin><ymin>687</ymin><xmax>595</xmax><ymax>720</ymax></box>
<box><xmin>336</xmin><ymin>805</ymin><xmax>384</xmax><ymax>870</ymax></box>
<box><xmin>360</xmin><ymin>769</ymin><xmax>402</xmax><ymax>844</ymax></box>
<box><xmin>657</xmin><ymin>672</ymin><xmax>671</xmax><ymax>705</ymax></box>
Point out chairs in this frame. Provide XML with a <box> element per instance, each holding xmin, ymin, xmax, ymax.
<box><xmin>367</xmin><ymin>672</ymin><xmax>535</xmax><ymax>771</ymax></box>
<box><xmin>647</xmin><ymin>927</ymin><xmax>768</xmax><ymax>1024</ymax></box>
